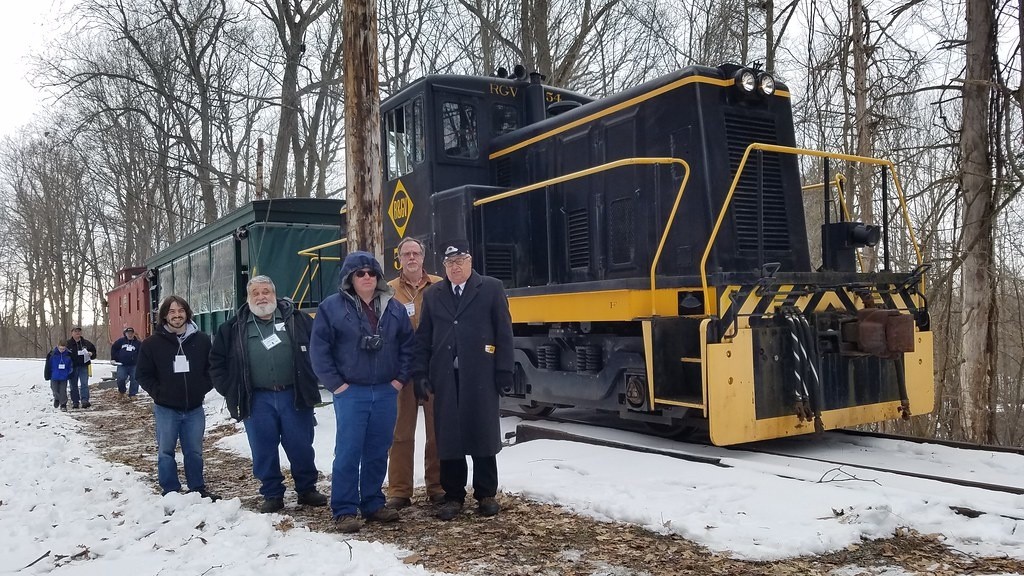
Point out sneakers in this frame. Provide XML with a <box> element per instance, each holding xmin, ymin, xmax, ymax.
<box><xmin>335</xmin><ymin>514</ymin><xmax>361</xmax><ymax>532</ymax></box>
<box><xmin>480</xmin><ymin>495</ymin><xmax>500</xmax><ymax>517</ymax></box>
<box><xmin>440</xmin><ymin>500</ymin><xmax>462</xmax><ymax>519</ymax></box>
<box><xmin>361</xmin><ymin>504</ymin><xmax>398</xmax><ymax>523</ymax></box>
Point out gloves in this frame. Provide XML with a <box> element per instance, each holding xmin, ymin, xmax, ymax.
<box><xmin>494</xmin><ymin>370</ymin><xmax>513</xmax><ymax>397</ymax></box>
<box><xmin>413</xmin><ymin>371</ymin><xmax>435</xmax><ymax>400</ymax></box>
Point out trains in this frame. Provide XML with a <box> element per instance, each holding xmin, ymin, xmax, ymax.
<box><xmin>105</xmin><ymin>64</ymin><xmax>935</xmax><ymax>448</ymax></box>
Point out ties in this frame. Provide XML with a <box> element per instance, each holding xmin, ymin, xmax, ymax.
<box><xmin>455</xmin><ymin>285</ymin><xmax>461</xmax><ymax>298</ymax></box>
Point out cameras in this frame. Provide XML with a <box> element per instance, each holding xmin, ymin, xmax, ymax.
<box><xmin>361</xmin><ymin>336</ymin><xmax>383</xmax><ymax>350</ymax></box>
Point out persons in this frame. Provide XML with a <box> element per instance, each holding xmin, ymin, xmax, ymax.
<box><xmin>44</xmin><ymin>339</ymin><xmax>74</xmax><ymax>412</ymax></box>
<box><xmin>386</xmin><ymin>237</ymin><xmax>447</xmax><ymax>509</ymax></box>
<box><xmin>412</xmin><ymin>240</ymin><xmax>515</xmax><ymax>522</ymax></box>
<box><xmin>111</xmin><ymin>327</ymin><xmax>141</xmax><ymax>401</ymax></box>
<box><xmin>66</xmin><ymin>327</ymin><xmax>96</xmax><ymax>408</ymax></box>
<box><xmin>309</xmin><ymin>251</ymin><xmax>416</xmax><ymax>533</ymax></box>
<box><xmin>208</xmin><ymin>274</ymin><xmax>328</xmax><ymax>514</ymax></box>
<box><xmin>136</xmin><ymin>296</ymin><xmax>222</xmax><ymax>504</ymax></box>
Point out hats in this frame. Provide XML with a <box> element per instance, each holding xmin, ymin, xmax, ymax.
<box><xmin>126</xmin><ymin>328</ymin><xmax>134</xmax><ymax>333</ymax></box>
<box><xmin>71</xmin><ymin>325</ymin><xmax>81</xmax><ymax>333</ymax></box>
<box><xmin>440</xmin><ymin>239</ymin><xmax>471</xmax><ymax>260</ymax></box>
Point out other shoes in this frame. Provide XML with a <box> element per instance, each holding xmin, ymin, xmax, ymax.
<box><xmin>387</xmin><ymin>497</ymin><xmax>412</xmax><ymax>509</ymax></box>
<box><xmin>61</xmin><ymin>403</ymin><xmax>67</xmax><ymax>411</ymax></box>
<box><xmin>430</xmin><ymin>493</ymin><xmax>445</xmax><ymax>506</ymax></box>
<box><xmin>192</xmin><ymin>487</ymin><xmax>223</xmax><ymax>502</ymax></box>
<box><xmin>73</xmin><ymin>402</ymin><xmax>79</xmax><ymax>408</ymax></box>
<box><xmin>261</xmin><ymin>495</ymin><xmax>284</xmax><ymax>513</ymax></box>
<box><xmin>82</xmin><ymin>403</ymin><xmax>91</xmax><ymax>408</ymax></box>
<box><xmin>131</xmin><ymin>395</ymin><xmax>137</xmax><ymax>401</ymax></box>
<box><xmin>54</xmin><ymin>399</ymin><xmax>59</xmax><ymax>408</ymax></box>
<box><xmin>298</xmin><ymin>489</ymin><xmax>329</xmax><ymax>506</ymax></box>
<box><xmin>162</xmin><ymin>488</ymin><xmax>178</xmax><ymax>497</ymax></box>
<box><xmin>120</xmin><ymin>392</ymin><xmax>127</xmax><ymax>400</ymax></box>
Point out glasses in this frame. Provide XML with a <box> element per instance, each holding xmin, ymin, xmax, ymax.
<box><xmin>443</xmin><ymin>255</ymin><xmax>470</xmax><ymax>269</ymax></box>
<box><xmin>352</xmin><ymin>270</ymin><xmax>378</xmax><ymax>278</ymax></box>
